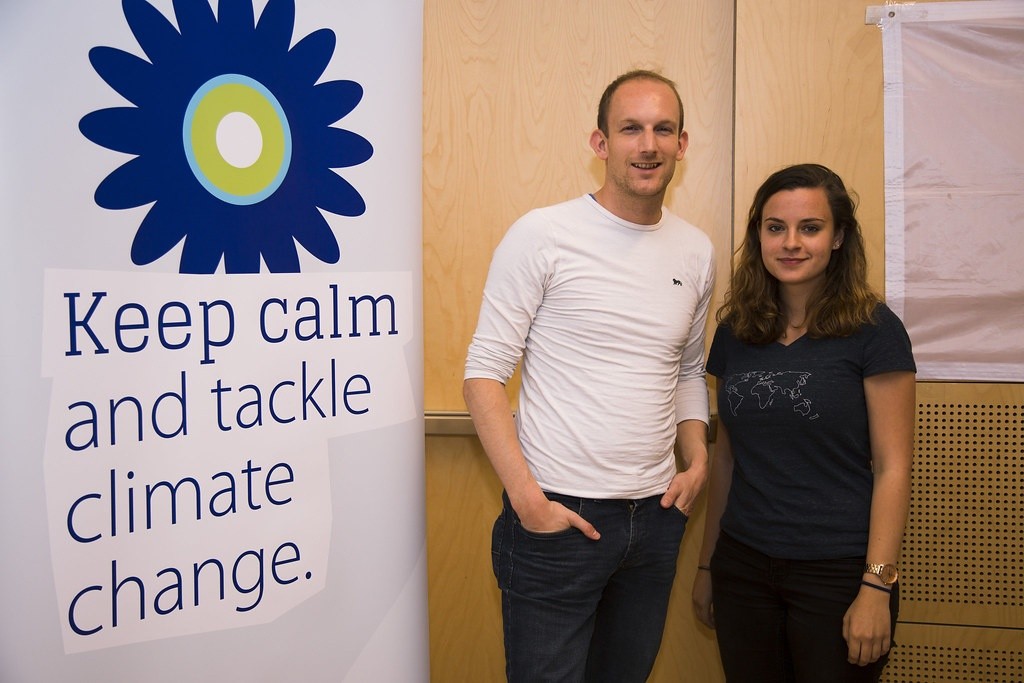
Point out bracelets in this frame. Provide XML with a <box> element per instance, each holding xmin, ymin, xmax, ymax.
<box><xmin>698</xmin><ymin>566</ymin><xmax>710</xmax><ymax>571</ymax></box>
<box><xmin>860</xmin><ymin>580</ymin><xmax>891</xmax><ymax>595</ymax></box>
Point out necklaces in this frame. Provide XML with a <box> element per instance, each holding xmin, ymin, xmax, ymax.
<box><xmin>588</xmin><ymin>193</ymin><xmax>596</xmax><ymax>203</ymax></box>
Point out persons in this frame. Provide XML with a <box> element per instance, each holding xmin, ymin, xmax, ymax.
<box><xmin>692</xmin><ymin>163</ymin><xmax>918</xmax><ymax>683</ymax></box>
<box><xmin>463</xmin><ymin>70</ymin><xmax>715</xmax><ymax>683</ymax></box>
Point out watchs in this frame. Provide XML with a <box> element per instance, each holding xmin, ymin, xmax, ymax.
<box><xmin>863</xmin><ymin>563</ymin><xmax>899</xmax><ymax>585</ymax></box>
